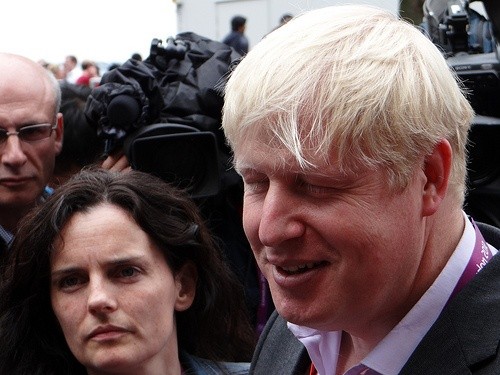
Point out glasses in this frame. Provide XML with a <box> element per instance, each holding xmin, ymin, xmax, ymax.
<box><xmin>0</xmin><ymin>114</ymin><xmax>58</xmax><ymax>144</ymax></box>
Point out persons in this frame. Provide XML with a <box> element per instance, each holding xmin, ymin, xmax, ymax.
<box><xmin>0</xmin><ymin>171</ymin><xmax>260</xmax><ymax>375</ymax></box>
<box><xmin>221</xmin><ymin>13</ymin><xmax>294</xmax><ymax>56</ymax></box>
<box><xmin>221</xmin><ymin>4</ymin><xmax>500</xmax><ymax>375</ymax></box>
<box><xmin>0</xmin><ymin>53</ymin><xmax>64</xmax><ymax>250</ymax></box>
<box><xmin>38</xmin><ymin>54</ymin><xmax>143</xmax><ymax>202</ymax></box>
